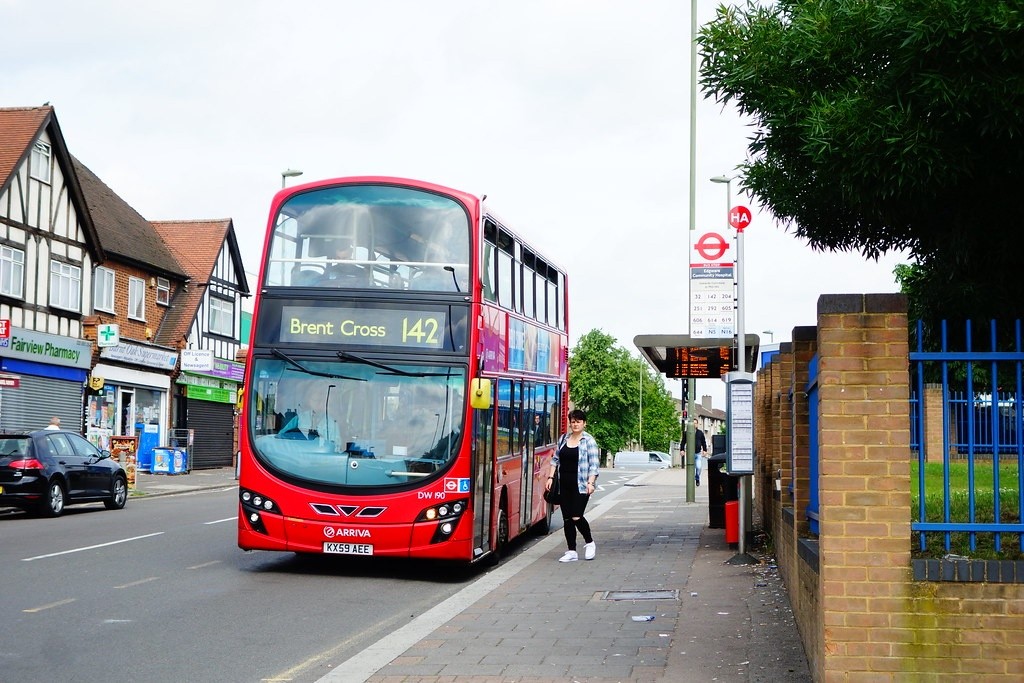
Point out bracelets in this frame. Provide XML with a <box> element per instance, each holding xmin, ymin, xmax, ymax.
<box><xmin>548</xmin><ymin>477</ymin><xmax>554</xmax><ymax>479</ymax></box>
<box><xmin>588</xmin><ymin>482</ymin><xmax>594</xmax><ymax>485</ymax></box>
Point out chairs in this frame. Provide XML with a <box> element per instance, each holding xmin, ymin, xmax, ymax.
<box><xmin>291</xmin><ymin>263</ymin><xmax>448</xmax><ymax>290</ymax></box>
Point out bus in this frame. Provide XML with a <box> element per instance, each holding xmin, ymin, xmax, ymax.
<box><xmin>237</xmin><ymin>176</ymin><xmax>570</xmax><ymax>566</ymax></box>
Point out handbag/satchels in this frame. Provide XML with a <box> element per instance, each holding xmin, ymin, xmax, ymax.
<box><xmin>544</xmin><ymin>472</ymin><xmax>560</xmax><ymax>505</ymax></box>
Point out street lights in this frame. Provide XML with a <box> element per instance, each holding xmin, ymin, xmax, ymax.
<box><xmin>325</xmin><ymin>385</ymin><xmax>336</xmax><ymax>441</ymax></box>
<box><xmin>763</xmin><ymin>331</ymin><xmax>774</xmax><ymax>343</ymax></box>
<box><xmin>443</xmin><ymin>266</ymin><xmax>461</xmax><ymax>292</ymax></box>
<box><xmin>281</xmin><ymin>170</ymin><xmax>304</xmax><ymax>286</ymax></box>
<box><xmin>430</xmin><ymin>414</ymin><xmax>440</xmax><ymax>450</ymax></box>
<box><xmin>710</xmin><ymin>175</ymin><xmax>730</xmax><ymax>229</ymax></box>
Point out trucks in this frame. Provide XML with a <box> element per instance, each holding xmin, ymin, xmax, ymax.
<box><xmin>614</xmin><ymin>451</ymin><xmax>672</xmax><ymax>470</ymax></box>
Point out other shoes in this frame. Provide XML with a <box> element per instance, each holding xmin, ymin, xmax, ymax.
<box><xmin>696</xmin><ymin>479</ymin><xmax>700</xmax><ymax>486</ymax></box>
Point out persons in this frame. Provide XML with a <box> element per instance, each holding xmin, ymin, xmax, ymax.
<box><xmin>680</xmin><ymin>419</ymin><xmax>707</xmax><ymax>486</ymax></box>
<box><xmin>279</xmin><ymin>394</ymin><xmax>342</xmax><ymax>447</ymax></box>
<box><xmin>534</xmin><ymin>415</ymin><xmax>541</xmax><ymax>447</ymax></box>
<box><xmin>545</xmin><ymin>410</ymin><xmax>600</xmax><ymax>562</ymax></box>
<box><xmin>329</xmin><ymin>245</ymin><xmax>364</xmax><ymax>278</ymax></box>
<box><xmin>45</xmin><ymin>417</ymin><xmax>66</xmax><ymax>448</ymax></box>
<box><xmin>98</xmin><ymin>436</ymin><xmax>102</xmax><ymax>452</ymax></box>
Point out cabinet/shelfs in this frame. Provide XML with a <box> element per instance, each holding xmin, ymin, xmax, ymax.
<box><xmin>169</xmin><ymin>428</ymin><xmax>194</xmax><ymax>474</ymax></box>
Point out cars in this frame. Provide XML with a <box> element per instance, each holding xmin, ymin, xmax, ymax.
<box><xmin>0</xmin><ymin>430</ymin><xmax>128</xmax><ymax>519</ymax></box>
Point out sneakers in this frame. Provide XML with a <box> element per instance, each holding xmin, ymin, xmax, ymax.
<box><xmin>583</xmin><ymin>541</ymin><xmax>596</xmax><ymax>559</ymax></box>
<box><xmin>559</xmin><ymin>549</ymin><xmax>579</xmax><ymax>562</ymax></box>
